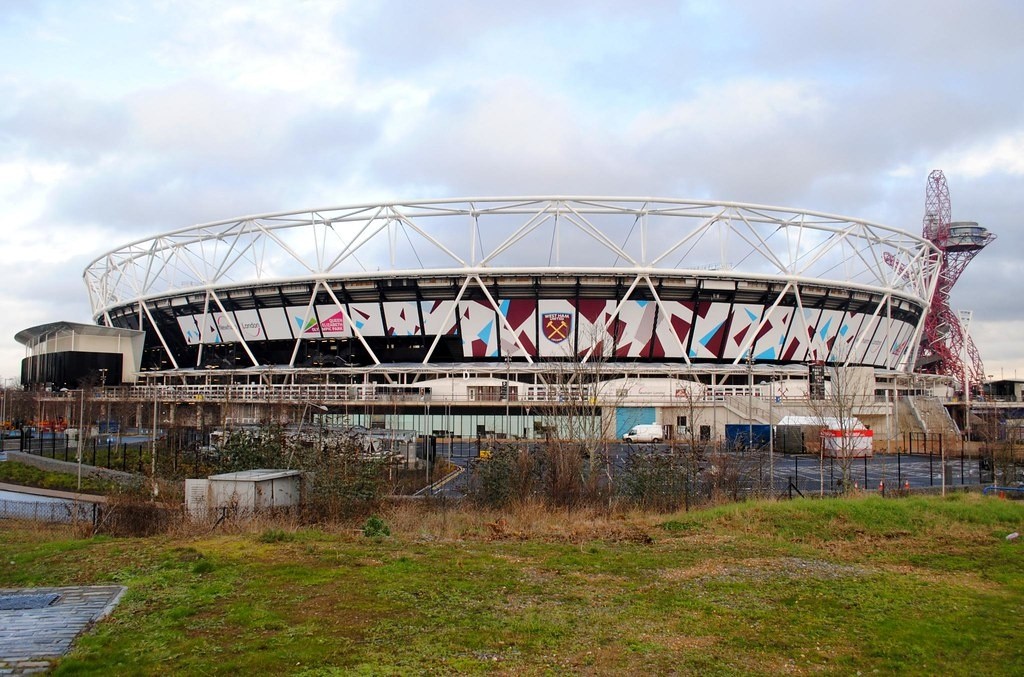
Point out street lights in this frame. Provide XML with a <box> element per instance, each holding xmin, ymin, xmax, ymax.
<box><xmin>149</xmin><ymin>367</ymin><xmax>161</xmax><ymax>387</ymax></box>
<box><xmin>777</xmin><ymin>380</ymin><xmax>786</xmax><ymax>421</ymax></box>
<box><xmin>205</xmin><ymin>365</ymin><xmax>220</xmax><ymax>401</ymax></box>
<box><xmin>525</xmin><ymin>408</ymin><xmax>530</xmax><ymax>462</ymax></box>
<box><xmin>760</xmin><ymin>380</ymin><xmax>774</xmax><ymax>495</ymax></box>
<box><xmin>59</xmin><ymin>387</ymin><xmax>88</xmax><ymax>491</ymax></box>
<box><xmin>425</xmin><ymin>402</ymin><xmax>432</xmax><ymax>484</ymax></box>
<box><xmin>98</xmin><ymin>368</ymin><xmax>108</xmax><ymax>394</ymax></box>
<box><xmin>286</xmin><ymin>400</ymin><xmax>328</xmax><ymax>471</ymax></box>
<box><xmin>987</xmin><ymin>375</ymin><xmax>994</xmax><ymax>402</ymax></box>
<box><xmin>0</xmin><ymin>378</ymin><xmax>14</xmax><ymax>426</ymax></box>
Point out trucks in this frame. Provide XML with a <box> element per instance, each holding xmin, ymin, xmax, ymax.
<box><xmin>622</xmin><ymin>424</ymin><xmax>664</xmax><ymax>444</ymax></box>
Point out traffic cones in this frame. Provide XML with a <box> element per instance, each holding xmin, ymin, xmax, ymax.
<box><xmin>998</xmin><ymin>490</ymin><xmax>1005</xmax><ymax>500</ymax></box>
<box><xmin>903</xmin><ymin>479</ymin><xmax>910</xmax><ymax>489</ymax></box>
<box><xmin>854</xmin><ymin>481</ymin><xmax>858</xmax><ymax>491</ymax></box>
<box><xmin>878</xmin><ymin>481</ymin><xmax>884</xmax><ymax>489</ymax></box>
